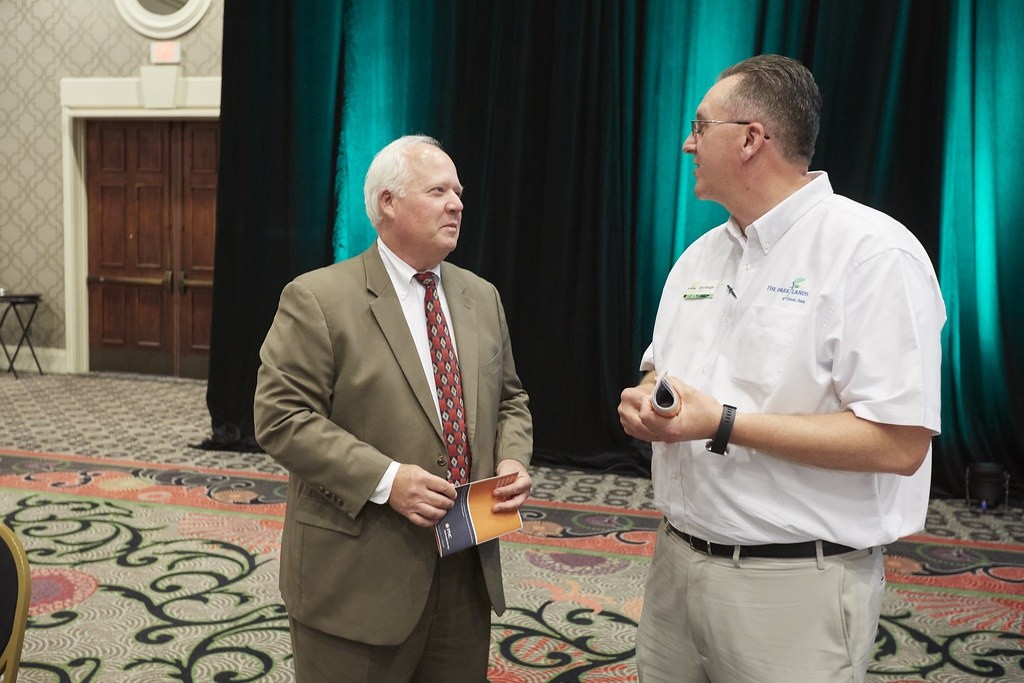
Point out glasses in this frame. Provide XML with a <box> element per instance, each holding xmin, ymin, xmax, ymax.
<box><xmin>691</xmin><ymin>119</ymin><xmax>770</xmax><ymax>140</ymax></box>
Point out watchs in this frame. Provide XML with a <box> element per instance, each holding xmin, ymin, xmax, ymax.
<box><xmin>705</xmin><ymin>404</ymin><xmax>737</xmax><ymax>455</ymax></box>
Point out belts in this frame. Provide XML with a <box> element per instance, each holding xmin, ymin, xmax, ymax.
<box><xmin>663</xmin><ymin>515</ymin><xmax>857</xmax><ymax>558</ymax></box>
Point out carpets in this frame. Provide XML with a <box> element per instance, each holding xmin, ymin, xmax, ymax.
<box><xmin>0</xmin><ymin>447</ymin><xmax>1024</xmax><ymax>683</ymax></box>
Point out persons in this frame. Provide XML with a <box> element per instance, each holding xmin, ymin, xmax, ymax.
<box><xmin>253</xmin><ymin>135</ymin><xmax>532</xmax><ymax>683</ymax></box>
<box><xmin>617</xmin><ymin>54</ymin><xmax>947</xmax><ymax>683</ymax></box>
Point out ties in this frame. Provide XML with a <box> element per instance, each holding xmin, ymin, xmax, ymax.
<box><xmin>413</xmin><ymin>271</ymin><xmax>472</xmax><ymax>488</ymax></box>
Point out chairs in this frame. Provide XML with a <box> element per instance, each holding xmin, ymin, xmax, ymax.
<box><xmin>0</xmin><ymin>522</ymin><xmax>31</xmax><ymax>683</ymax></box>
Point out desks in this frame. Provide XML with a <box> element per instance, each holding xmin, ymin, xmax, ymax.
<box><xmin>0</xmin><ymin>297</ymin><xmax>43</xmax><ymax>380</ymax></box>
